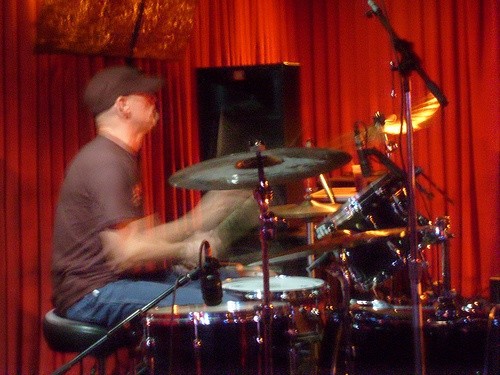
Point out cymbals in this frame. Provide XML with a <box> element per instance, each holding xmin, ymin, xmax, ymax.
<box><xmin>167</xmin><ymin>146</ymin><xmax>354</xmax><ymax>188</ymax></box>
<box><xmin>266</xmin><ymin>202</ymin><xmax>340</xmax><ymax>217</ymax></box>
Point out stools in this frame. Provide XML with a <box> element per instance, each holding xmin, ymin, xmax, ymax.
<box><xmin>43</xmin><ymin>307</ymin><xmax>131</xmax><ymax>375</ymax></box>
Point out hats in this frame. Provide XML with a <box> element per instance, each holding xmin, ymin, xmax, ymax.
<box><xmin>85</xmin><ymin>65</ymin><xmax>164</xmax><ymax>118</ymax></box>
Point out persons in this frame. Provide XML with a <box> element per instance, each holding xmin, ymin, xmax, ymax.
<box><xmin>51</xmin><ymin>64</ymin><xmax>312</xmax><ymax>375</ymax></box>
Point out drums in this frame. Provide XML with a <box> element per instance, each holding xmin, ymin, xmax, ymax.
<box><xmin>146</xmin><ymin>301</ymin><xmax>292</xmax><ymax>374</ymax></box>
<box><xmin>343</xmin><ymin>299</ymin><xmax>500</xmax><ymax>374</ymax></box>
<box><xmin>221</xmin><ymin>275</ymin><xmax>328</xmax><ymax>339</ymax></box>
<box><xmin>306</xmin><ymin>188</ymin><xmax>357</xmax><ymax>204</ymax></box>
<box><xmin>313</xmin><ymin>168</ymin><xmax>438</xmax><ymax>290</ymax></box>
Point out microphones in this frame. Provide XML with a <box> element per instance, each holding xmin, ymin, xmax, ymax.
<box><xmin>199</xmin><ymin>242</ymin><xmax>224</xmax><ymax>307</ymax></box>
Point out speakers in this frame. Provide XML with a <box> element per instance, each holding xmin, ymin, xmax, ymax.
<box><xmin>194</xmin><ymin>63</ymin><xmax>305</xmax><ymax>234</ymax></box>
<box><xmin>354</xmin><ymin>124</ymin><xmax>372</xmax><ymax>177</ymax></box>
